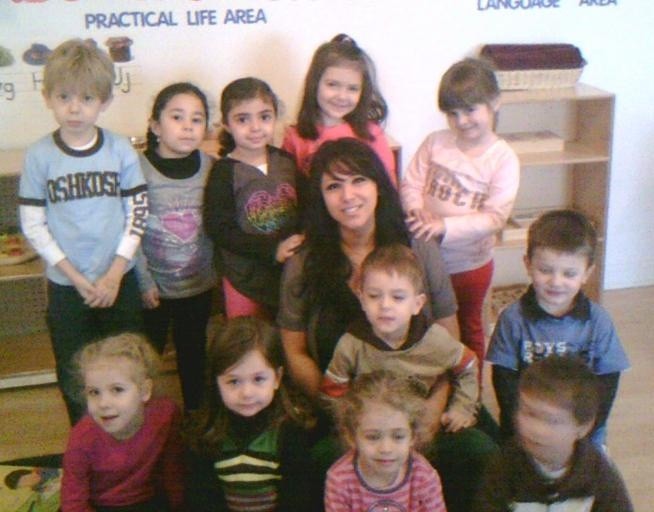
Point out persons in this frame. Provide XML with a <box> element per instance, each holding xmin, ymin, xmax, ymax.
<box><xmin>57</xmin><ymin>330</ymin><xmax>187</xmax><ymax>511</ymax></box>
<box><xmin>15</xmin><ymin>36</ymin><xmax>150</xmax><ymax>426</ymax></box>
<box><xmin>466</xmin><ymin>350</ymin><xmax>636</xmax><ymax>511</ymax></box>
<box><xmin>129</xmin><ymin>80</ymin><xmax>218</xmax><ymax>425</ymax></box>
<box><xmin>482</xmin><ymin>207</ymin><xmax>632</xmax><ymax>465</ymax></box>
<box><xmin>319</xmin><ymin>368</ymin><xmax>448</xmax><ymax>512</ymax></box>
<box><xmin>325</xmin><ymin>241</ymin><xmax>483</xmax><ymax>437</ymax></box>
<box><xmin>201</xmin><ymin>76</ymin><xmax>307</xmax><ymax>322</ymax></box>
<box><xmin>397</xmin><ymin>58</ymin><xmax>520</xmax><ymax>358</ymax></box>
<box><xmin>277</xmin><ymin>137</ymin><xmax>504</xmax><ymax>441</ymax></box>
<box><xmin>4</xmin><ymin>467</ymin><xmax>63</xmax><ymax>493</ymax></box>
<box><xmin>277</xmin><ymin>33</ymin><xmax>398</xmax><ymax>192</ymax></box>
<box><xmin>184</xmin><ymin>314</ymin><xmax>313</xmax><ymax>512</ymax></box>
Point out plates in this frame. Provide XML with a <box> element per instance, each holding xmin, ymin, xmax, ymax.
<box><xmin>0</xmin><ymin>233</ymin><xmax>40</xmax><ymax>267</ymax></box>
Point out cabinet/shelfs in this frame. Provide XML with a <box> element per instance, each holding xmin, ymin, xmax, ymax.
<box><xmin>0</xmin><ymin>120</ymin><xmax>402</xmax><ymax>389</ymax></box>
<box><xmin>487</xmin><ymin>82</ymin><xmax>616</xmax><ymax>336</ymax></box>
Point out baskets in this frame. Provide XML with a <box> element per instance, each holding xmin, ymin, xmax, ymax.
<box><xmin>494</xmin><ymin>59</ymin><xmax>584</xmax><ymax>90</ymax></box>
